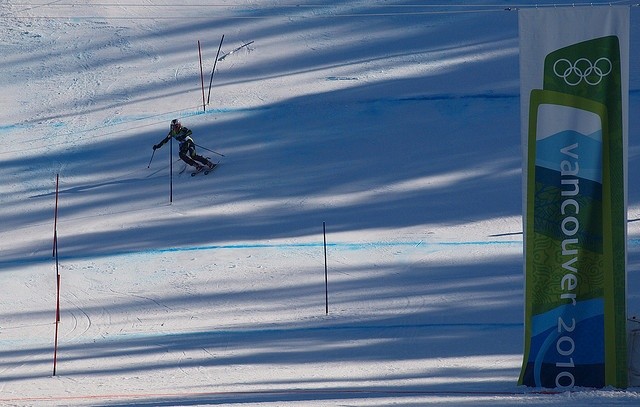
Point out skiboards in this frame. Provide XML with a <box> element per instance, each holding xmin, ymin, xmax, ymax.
<box><xmin>191</xmin><ymin>157</ymin><xmax>222</xmax><ymax>178</ymax></box>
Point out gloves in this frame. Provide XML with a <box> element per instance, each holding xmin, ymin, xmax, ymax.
<box><xmin>176</xmin><ymin>136</ymin><xmax>183</xmax><ymax>141</ymax></box>
<box><xmin>153</xmin><ymin>145</ymin><xmax>157</xmax><ymax>150</ymax></box>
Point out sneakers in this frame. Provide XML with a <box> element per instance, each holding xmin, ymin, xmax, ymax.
<box><xmin>207</xmin><ymin>162</ymin><xmax>214</xmax><ymax>170</ymax></box>
<box><xmin>196</xmin><ymin>164</ymin><xmax>203</xmax><ymax>172</ymax></box>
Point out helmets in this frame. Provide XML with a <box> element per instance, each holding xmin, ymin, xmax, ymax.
<box><xmin>170</xmin><ymin>119</ymin><xmax>181</xmax><ymax>132</ymax></box>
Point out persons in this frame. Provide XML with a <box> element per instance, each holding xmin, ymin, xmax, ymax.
<box><xmin>152</xmin><ymin>119</ymin><xmax>216</xmax><ymax>170</ymax></box>
